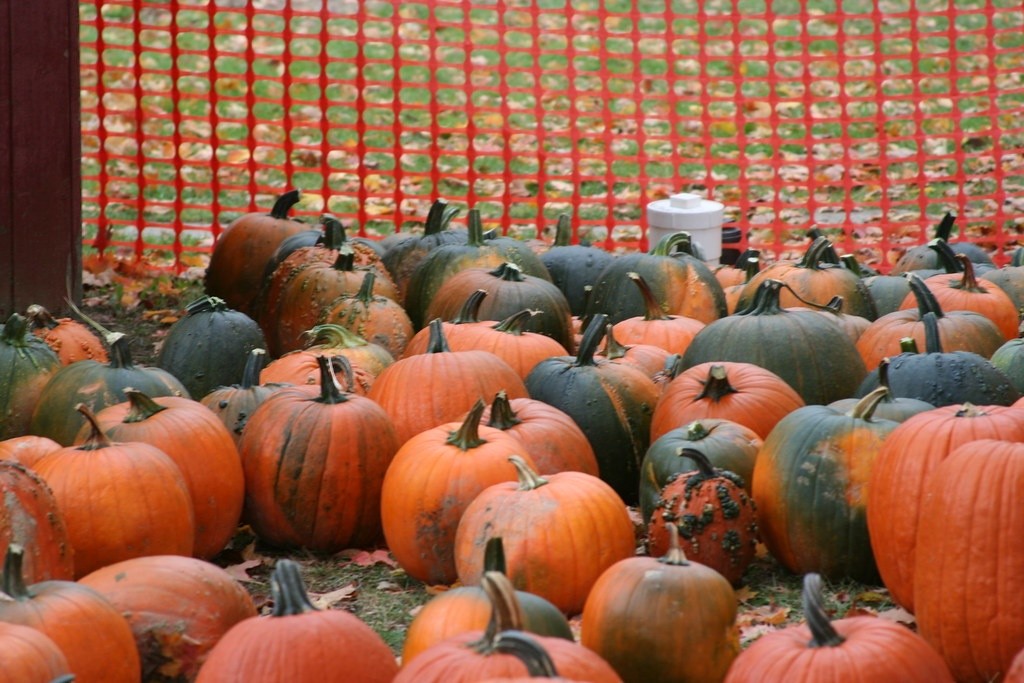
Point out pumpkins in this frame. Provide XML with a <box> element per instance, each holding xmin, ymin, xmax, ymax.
<box><xmin>0</xmin><ymin>189</ymin><xmax>1024</xmax><ymax>683</ymax></box>
